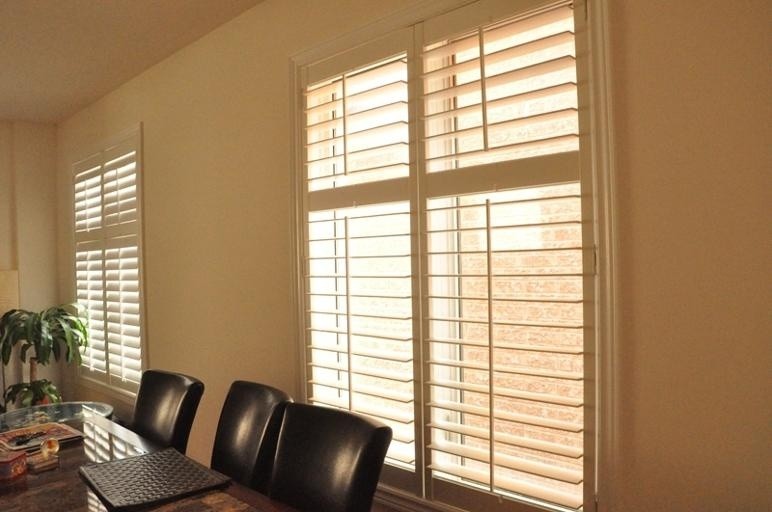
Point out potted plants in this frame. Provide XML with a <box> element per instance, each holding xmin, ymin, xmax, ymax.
<box><xmin>1</xmin><ymin>303</ymin><xmax>90</xmax><ymax>408</ymax></box>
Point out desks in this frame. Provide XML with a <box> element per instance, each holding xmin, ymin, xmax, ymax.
<box><xmin>0</xmin><ymin>402</ymin><xmax>301</xmax><ymax>512</ymax></box>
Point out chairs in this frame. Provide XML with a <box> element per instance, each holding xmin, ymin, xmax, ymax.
<box><xmin>125</xmin><ymin>369</ymin><xmax>393</xmax><ymax>511</ymax></box>
